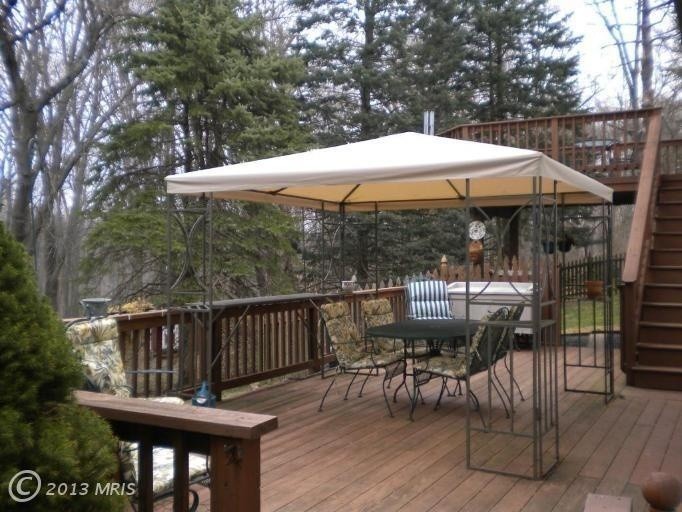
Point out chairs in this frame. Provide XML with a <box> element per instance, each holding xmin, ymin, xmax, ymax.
<box><xmin>588</xmin><ymin>127</ymin><xmax>645</xmax><ymax>177</ymax></box>
<box><xmin>65</xmin><ymin>314</ymin><xmax>212</xmax><ymax>506</ymax></box>
<box><xmin>316</xmin><ymin>276</ymin><xmax>526</xmax><ymax>432</ymax></box>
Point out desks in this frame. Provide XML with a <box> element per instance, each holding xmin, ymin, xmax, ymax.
<box><xmin>572</xmin><ymin>136</ymin><xmax>620</xmax><ymax>177</ymax></box>
